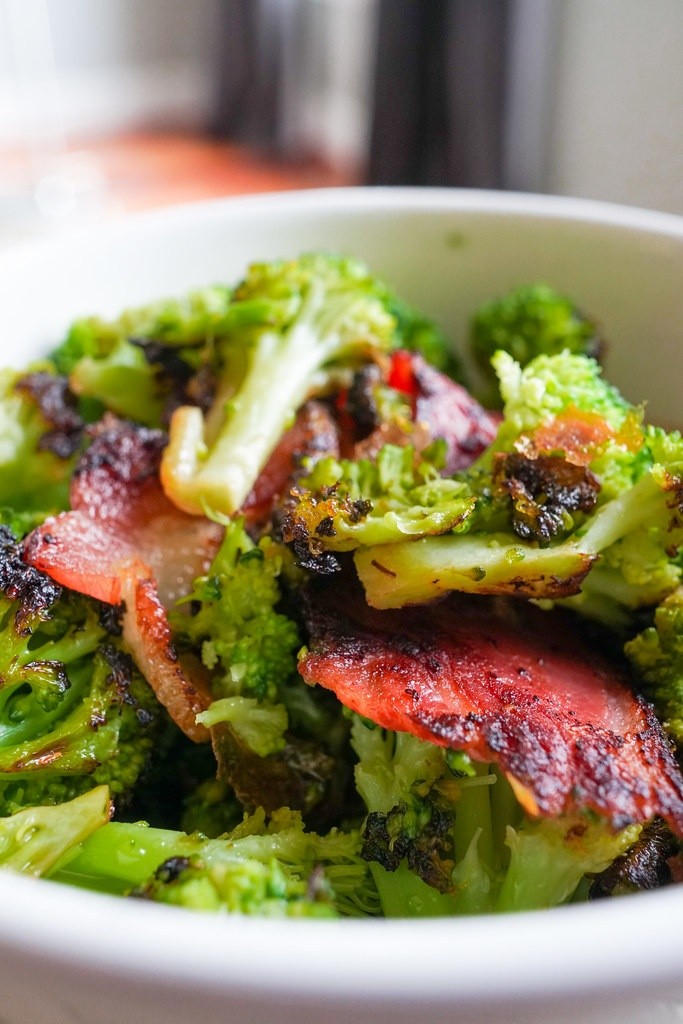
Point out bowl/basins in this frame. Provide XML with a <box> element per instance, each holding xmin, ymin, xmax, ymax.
<box><xmin>0</xmin><ymin>186</ymin><xmax>683</xmax><ymax>1022</ymax></box>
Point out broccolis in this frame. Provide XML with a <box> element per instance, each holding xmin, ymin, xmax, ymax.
<box><xmin>0</xmin><ymin>253</ymin><xmax>682</xmax><ymax>915</ymax></box>
